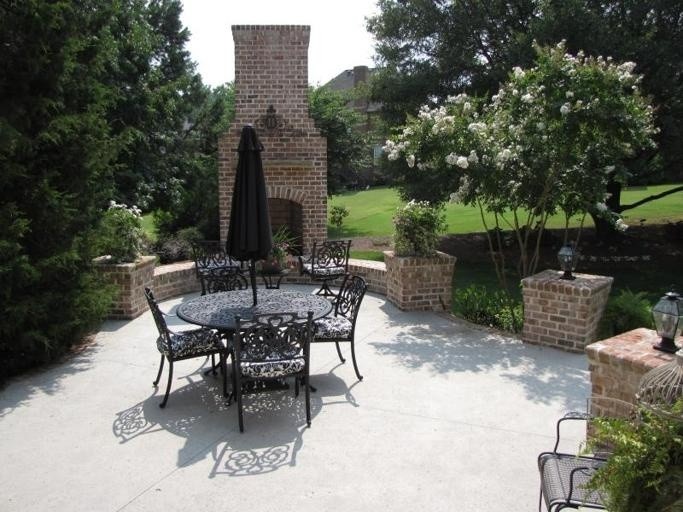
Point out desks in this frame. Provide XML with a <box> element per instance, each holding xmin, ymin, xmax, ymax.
<box><xmin>176</xmin><ymin>289</ymin><xmax>333</xmax><ymax>407</ymax></box>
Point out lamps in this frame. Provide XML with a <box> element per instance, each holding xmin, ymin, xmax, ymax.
<box><xmin>650</xmin><ymin>287</ymin><xmax>683</xmax><ymax>353</ymax></box>
<box><xmin>556</xmin><ymin>243</ymin><xmax>578</xmax><ymax>281</ymax></box>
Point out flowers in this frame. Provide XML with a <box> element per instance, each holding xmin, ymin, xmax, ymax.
<box><xmin>392</xmin><ymin>200</ymin><xmax>448</xmax><ymax>258</ymax></box>
<box><xmin>96</xmin><ymin>200</ymin><xmax>143</xmax><ymax>263</ymax></box>
<box><xmin>270</xmin><ymin>224</ymin><xmax>302</xmax><ymax>270</ymax></box>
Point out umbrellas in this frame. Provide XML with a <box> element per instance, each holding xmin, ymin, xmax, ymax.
<box><xmin>225</xmin><ymin>122</ymin><xmax>272</xmax><ymax>305</ymax></box>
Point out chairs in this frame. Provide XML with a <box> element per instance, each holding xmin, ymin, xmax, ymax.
<box><xmin>146</xmin><ymin>288</ymin><xmax>229</xmax><ymax>409</ymax></box>
<box><xmin>231</xmin><ymin>311</ymin><xmax>314</xmax><ymax>431</ymax></box>
<box><xmin>538</xmin><ymin>409</ymin><xmax>636</xmax><ymax>512</ymax></box>
<box><xmin>191</xmin><ymin>238</ymin><xmax>250</xmax><ymax>296</ymax></box>
<box><xmin>310</xmin><ymin>274</ymin><xmax>363</xmax><ymax>381</ymax></box>
<box><xmin>299</xmin><ymin>239</ymin><xmax>352</xmax><ymax>298</ymax></box>
